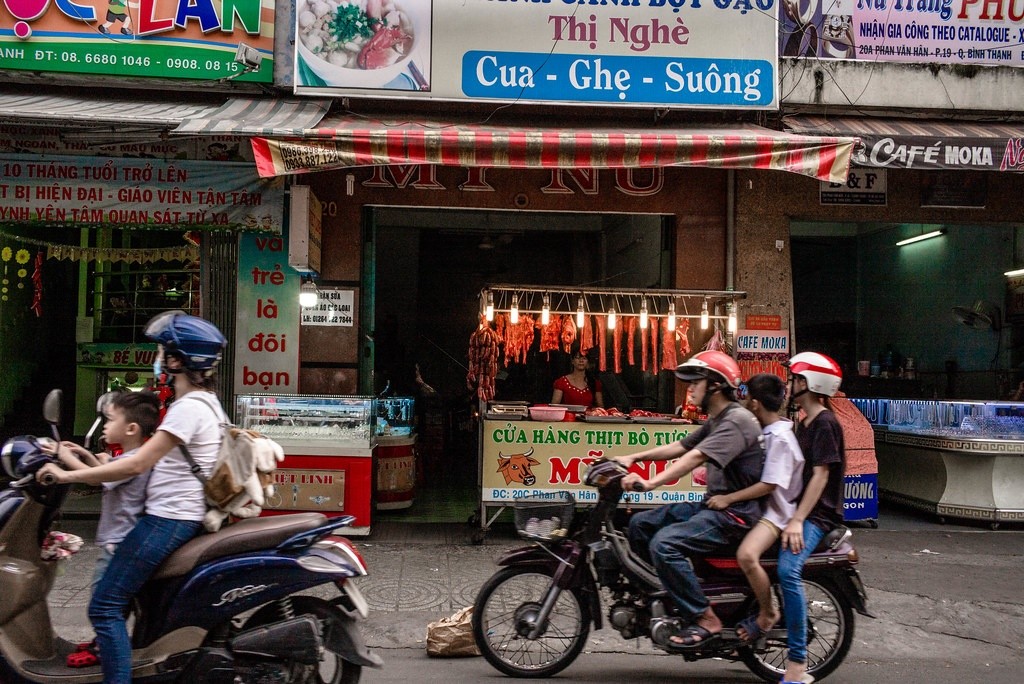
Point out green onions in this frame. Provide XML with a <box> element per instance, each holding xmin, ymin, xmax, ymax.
<box><xmin>312</xmin><ymin>3</ymin><xmax>388</xmax><ymax>54</ymax></box>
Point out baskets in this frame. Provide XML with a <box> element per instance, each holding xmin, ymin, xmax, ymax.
<box><xmin>514</xmin><ymin>491</ymin><xmax>583</xmax><ymax>541</ymax></box>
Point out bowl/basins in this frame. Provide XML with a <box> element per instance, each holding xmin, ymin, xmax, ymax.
<box><xmin>529</xmin><ymin>407</ymin><xmax>568</xmax><ymax>421</ymax></box>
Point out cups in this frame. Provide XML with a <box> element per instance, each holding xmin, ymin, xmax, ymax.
<box><xmin>872</xmin><ymin>366</ymin><xmax>880</xmax><ymax>375</ymax></box>
<box><xmin>858</xmin><ymin>361</ymin><xmax>870</xmax><ymax>376</ymax></box>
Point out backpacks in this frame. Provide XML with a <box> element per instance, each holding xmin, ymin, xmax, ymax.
<box><xmin>177</xmin><ymin>396</ymin><xmax>285</xmax><ymax>532</ymax></box>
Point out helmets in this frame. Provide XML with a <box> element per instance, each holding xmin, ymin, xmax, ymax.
<box><xmin>780</xmin><ymin>351</ymin><xmax>842</xmax><ymax>397</ymax></box>
<box><xmin>673</xmin><ymin>350</ymin><xmax>741</xmax><ymax>390</ymax></box>
<box><xmin>143</xmin><ymin>310</ymin><xmax>228</xmax><ymax>373</ymax></box>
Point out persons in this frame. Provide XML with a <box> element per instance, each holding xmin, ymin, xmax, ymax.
<box><xmin>39</xmin><ymin>392</ymin><xmax>161</xmax><ymax>667</ymax></box>
<box><xmin>707</xmin><ymin>372</ymin><xmax>805</xmax><ymax>642</ymax></box>
<box><xmin>552</xmin><ymin>349</ymin><xmax>603</xmax><ymax>408</ymax></box>
<box><xmin>611</xmin><ymin>349</ymin><xmax>764</xmax><ymax>649</ymax></box>
<box><xmin>777</xmin><ymin>351</ymin><xmax>846</xmax><ymax>684</ymax></box>
<box><xmin>1010</xmin><ymin>362</ymin><xmax>1024</xmax><ymax>402</ymax></box>
<box><xmin>37</xmin><ymin>310</ymin><xmax>229</xmax><ymax>684</ymax></box>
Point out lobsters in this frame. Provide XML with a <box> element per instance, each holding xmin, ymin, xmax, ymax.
<box><xmin>357</xmin><ymin>28</ymin><xmax>408</xmax><ymax>69</ymax></box>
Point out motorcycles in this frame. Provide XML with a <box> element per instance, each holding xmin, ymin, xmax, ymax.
<box><xmin>470</xmin><ymin>454</ymin><xmax>876</xmax><ymax>683</ymax></box>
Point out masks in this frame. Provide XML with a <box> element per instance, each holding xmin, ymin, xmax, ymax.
<box><xmin>153</xmin><ymin>360</ymin><xmax>175</xmax><ymax>384</ymax></box>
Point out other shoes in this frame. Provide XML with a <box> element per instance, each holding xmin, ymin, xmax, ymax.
<box><xmin>66</xmin><ymin>649</ymin><xmax>100</xmax><ymax>668</ymax></box>
<box><xmin>76</xmin><ymin>642</ymin><xmax>100</xmax><ymax>654</ymax></box>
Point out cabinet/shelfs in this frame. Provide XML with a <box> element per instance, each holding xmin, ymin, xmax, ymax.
<box><xmin>238</xmin><ymin>393</ymin><xmax>377</xmax><ymax>449</ymax></box>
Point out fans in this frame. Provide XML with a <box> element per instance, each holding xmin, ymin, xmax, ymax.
<box><xmin>952</xmin><ymin>295</ymin><xmax>1001</xmax><ymax>330</ymax></box>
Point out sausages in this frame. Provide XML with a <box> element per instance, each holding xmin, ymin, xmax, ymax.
<box><xmin>466</xmin><ymin>327</ymin><xmax>499</xmax><ymax>401</ymax></box>
<box><xmin>594</xmin><ymin>315</ymin><xmax>660</xmax><ymax>377</ymax></box>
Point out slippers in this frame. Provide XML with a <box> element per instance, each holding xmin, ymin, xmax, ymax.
<box><xmin>734</xmin><ymin>614</ymin><xmax>771</xmax><ymax>644</ymax></box>
<box><xmin>667</xmin><ymin>624</ymin><xmax>721</xmax><ymax>647</ymax></box>
<box><xmin>781</xmin><ymin>673</ymin><xmax>815</xmax><ymax>684</ymax></box>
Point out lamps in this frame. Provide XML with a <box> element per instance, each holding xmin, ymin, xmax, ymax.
<box><xmin>217</xmin><ymin>41</ymin><xmax>262</xmax><ymax>83</ymax></box>
<box><xmin>477</xmin><ymin>210</ymin><xmax>496</xmax><ymax>249</ymax></box>
<box><xmin>300</xmin><ymin>273</ymin><xmax>318</xmax><ymax>308</ymax></box>
<box><xmin>895</xmin><ymin>228</ymin><xmax>946</xmax><ymax>246</ymax></box>
<box><xmin>485</xmin><ymin>290</ymin><xmax>738</xmax><ymax>332</ymax></box>
<box><xmin>346</xmin><ymin>167</ymin><xmax>354</xmax><ymax>195</ymax></box>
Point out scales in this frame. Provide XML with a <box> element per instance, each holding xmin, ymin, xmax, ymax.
<box><xmin>487</xmin><ymin>400</ymin><xmax>530</xmax><ymax>418</ymax></box>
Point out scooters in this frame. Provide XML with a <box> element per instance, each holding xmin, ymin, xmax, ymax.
<box><xmin>0</xmin><ymin>388</ymin><xmax>387</xmax><ymax>684</ymax></box>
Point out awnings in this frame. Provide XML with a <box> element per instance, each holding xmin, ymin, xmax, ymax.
<box><xmin>0</xmin><ymin>87</ymin><xmax>859</xmax><ymax>186</ymax></box>
<box><xmin>782</xmin><ymin>116</ymin><xmax>1024</xmax><ymax>172</ymax></box>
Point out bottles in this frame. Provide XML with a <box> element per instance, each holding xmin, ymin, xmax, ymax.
<box><xmin>881</xmin><ymin>345</ymin><xmax>918</xmax><ymax>380</ymax></box>
<box><xmin>370</xmin><ymin>424</ymin><xmax>382</xmax><ymax>437</ymax></box>
<box><xmin>384</xmin><ymin>424</ymin><xmax>390</xmax><ymax>436</ymax></box>
